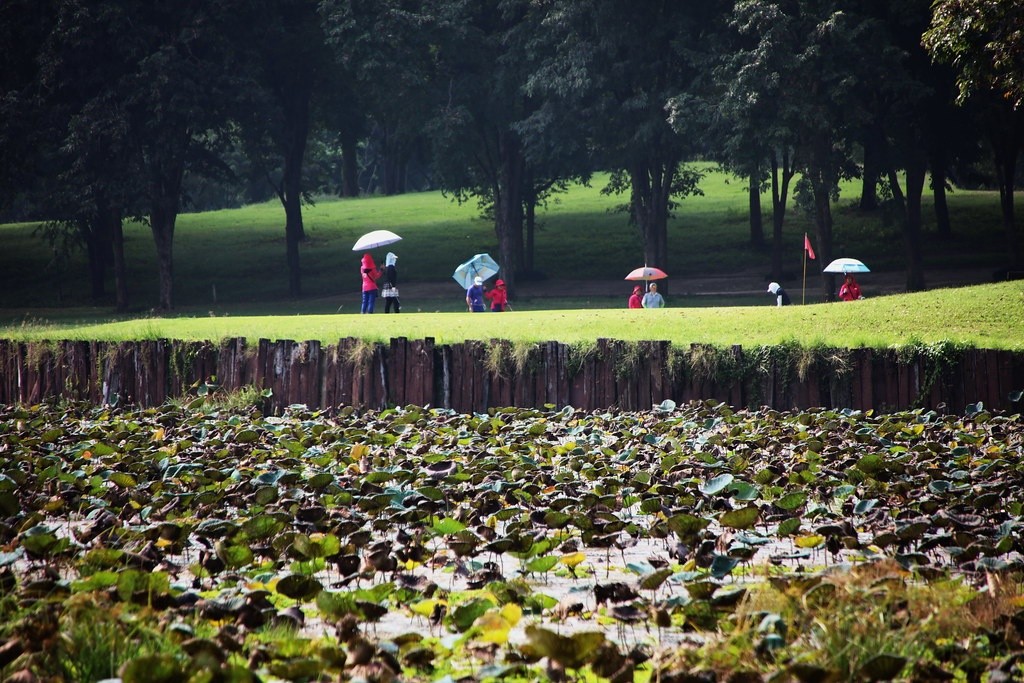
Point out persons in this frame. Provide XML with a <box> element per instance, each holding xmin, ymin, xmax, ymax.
<box><xmin>382</xmin><ymin>252</ymin><xmax>401</xmax><ymax>313</ymax></box>
<box><xmin>839</xmin><ymin>274</ymin><xmax>861</xmax><ymax>301</ymax></box>
<box><xmin>361</xmin><ymin>254</ymin><xmax>385</xmax><ymax>314</ymax></box>
<box><xmin>466</xmin><ymin>277</ymin><xmax>485</xmax><ymax>312</ymax></box>
<box><xmin>642</xmin><ymin>283</ymin><xmax>664</xmax><ymax>309</ymax></box>
<box><xmin>629</xmin><ymin>285</ymin><xmax>644</xmax><ymax>309</ymax></box>
<box><xmin>767</xmin><ymin>282</ymin><xmax>793</xmax><ymax>306</ymax></box>
<box><xmin>483</xmin><ymin>280</ymin><xmax>507</xmax><ymax>312</ymax></box>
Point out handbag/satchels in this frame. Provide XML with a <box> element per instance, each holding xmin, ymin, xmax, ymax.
<box><xmin>382</xmin><ymin>285</ymin><xmax>400</xmax><ymax>298</ymax></box>
<box><xmin>494</xmin><ymin>303</ymin><xmax>502</xmax><ymax>312</ymax></box>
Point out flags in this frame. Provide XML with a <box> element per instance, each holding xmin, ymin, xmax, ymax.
<box><xmin>805</xmin><ymin>235</ymin><xmax>815</xmax><ymax>259</ymax></box>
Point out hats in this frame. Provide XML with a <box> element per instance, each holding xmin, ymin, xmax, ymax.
<box><xmin>474</xmin><ymin>276</ymin><xmax>482</xmax><ymax>285</ymax></box>
<box><xmin>385</xmin><ymin>252</ymin><xmax>398</xmax><ymax>267</ymax></box>
<box><xmin>767</xmin><ymin>282</ymin><xmax>780</xmax><ymax>295</ymax></box>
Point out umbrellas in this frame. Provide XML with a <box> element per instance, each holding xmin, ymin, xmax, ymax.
<box><xmin>452</xmin><ymin>254</ymin><xmax>499</xmax><ymax>289</ymax></box>
<box><xmin>823</xmin><ymin>258</ymin><xmax>870</xmax><ymax>287</ymax></box>
<box><xmin>625</xmin><ymin>263</ymin><xmax>668</xmax><ymax>292</ymax></box>
<box><xmin>352</xmin><ymin>230</ymin><xmax>402</xmax><ymax>265</ymax></box>
